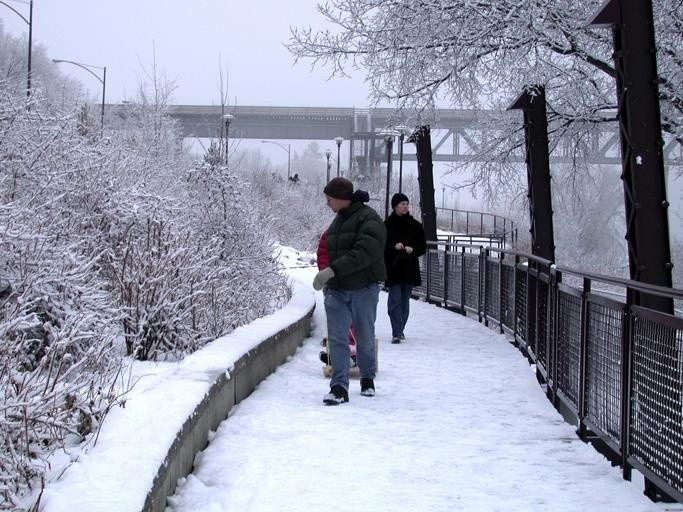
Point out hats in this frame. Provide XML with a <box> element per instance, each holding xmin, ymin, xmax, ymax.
<box><xmin>323</xmin><ymin>177</ymin><xmax>353</xmax><ymax>199</ymax></box>
<box><xmin>390</xmin><ymin>193</ymin><xmax>409</xmax><ymax>209</ymax></box>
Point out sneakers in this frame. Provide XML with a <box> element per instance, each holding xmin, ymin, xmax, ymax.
<box><xmin>391</xmin><ymin>334</ymin><xmax>404</xmax><ymax>344</ymax></box>
<box><xmin>322</xmin><ymin>385</ymin><xmax>348</xmax><ymax>405</ymax></box>
<box><xmin>359</xmin><ymin>378</ymin><xmax>374</xmax><ymax>396</ymax></box>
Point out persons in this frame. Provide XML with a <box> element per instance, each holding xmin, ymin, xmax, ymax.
<box><xmin>311</xmin><ymin>176</ymin><xmax>388</xmax><ymax>405</ymax></box>
<box><xmin>384</xmin><ymin>193</ymin><xmax>427</xmax><ymax>344</ymax></box>
<box><xmin>318</xmin><ymin>323</ymin><xmax>357</xmax><ymax>368</ymax></box>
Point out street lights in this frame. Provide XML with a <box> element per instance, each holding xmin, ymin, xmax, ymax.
<box><xmin>326</xmin><ymin>136</ymin><xmax>345</xmax><ymax>185</ymax></box>
<box><xmin>220</xmin><ymin>114</ymin><xmax>236</xmax><ymax>165</ymax></box>
<box><xmin>53</xmin><ymin>59</ymin><xmax>106</xmax><ymax>138</ymax></box>
<box><xmin>262</xmin><ymin>141</ymin><xmax>290</xmax><ymax>179</ymax></box>
<box><xmin>377</xmin><ymin>126</ymin><xmax>409</xmax><ymax>220</ymax></box>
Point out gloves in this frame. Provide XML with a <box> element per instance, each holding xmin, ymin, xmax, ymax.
<box><xmin>312</xmin><ymin>267</ymin><xmax>335</xmax><ymax>290</ymax></box>
